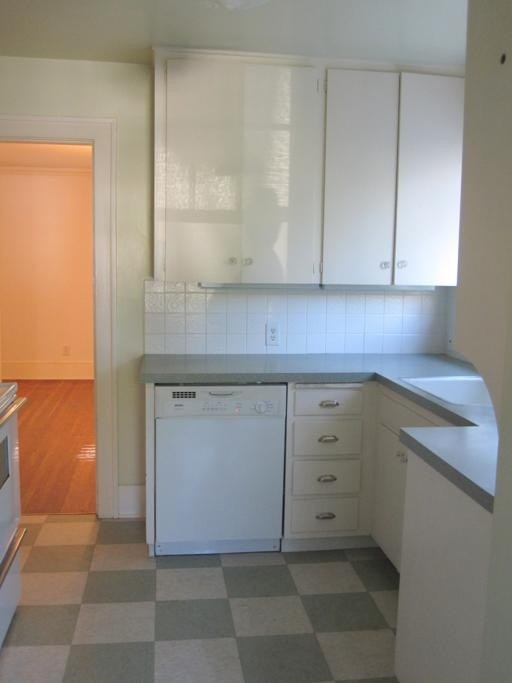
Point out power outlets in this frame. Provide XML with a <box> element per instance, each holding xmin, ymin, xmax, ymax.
<box><xmin>265</xmin><ymin>323</ymin><xmax>281</xmax><ymax>347</ymax></box>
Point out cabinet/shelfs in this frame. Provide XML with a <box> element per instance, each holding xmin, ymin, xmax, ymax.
<box><xmin>149</xmin><ymin>45</ymin><xmax>465</xmax><ymax>289</ymax></box>
<box><xmin>280</xmin><ymin>382</ymin><xmax>375</xmax><ymax>554</ymax></box>
<box><xmin>368</xmin><ymin>378</ymin><xmax>455</xmax><ymax>575</ymax></box>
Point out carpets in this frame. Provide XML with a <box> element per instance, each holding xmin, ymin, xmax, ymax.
<box><xmin>0</xmin><ymin>512</ymin><xmax>398</xmax><ymax>683</ymax></box>
